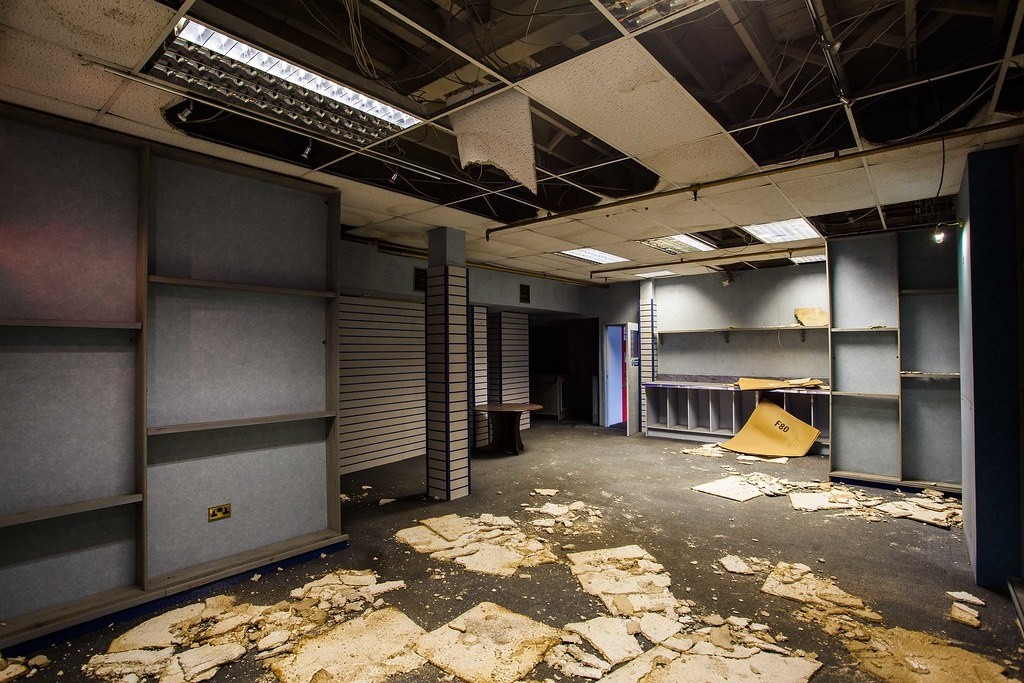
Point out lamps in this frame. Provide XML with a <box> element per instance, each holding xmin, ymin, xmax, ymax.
<box><xmin>301</xmin><ymin>137</ymin><xmax>312</xmax><ymax>159</ymax></box>
<box><xmin>176</xmin><ymin>99</ymin><xmax>193</xmax><ymax>122</ymax></box>
<box><xmin>388</xmin><ymin>165</ymin><xmax>399</xmax><ymax>184</ymax></box>
<box><xmin>817</xmin><ymin>38</ymin><xmax>841</xmax><ymax>57</ymax></box>
<box><xmin>934</xmin><ymin>217</ymin><xmax>963</xmax><ymax>245</ymax></box>
<box><xmin>833</xmin><ymin>92</ymin><xmax>855</xmax><ymax>108</ymax></box>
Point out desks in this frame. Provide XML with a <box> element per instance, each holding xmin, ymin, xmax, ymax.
<box><xmin>473</xmin><ymin>403</ymin><xmax>543</xmax><ymax>457</ymax></box>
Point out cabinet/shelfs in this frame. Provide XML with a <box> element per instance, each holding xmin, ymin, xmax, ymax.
<box><xmin>822</xmin><ymin>229</ymin><xmax>963</xmax><ymax>495</ymax></box>
<box><xmin>0</xmin><ymin>98</ymin><xmax>351</xmax><ymax>653</ymax></box>
<box><xmin>642</xmin><ymin>385</ymin><xmax>831</xmax><ymax>454</ymax></box>
